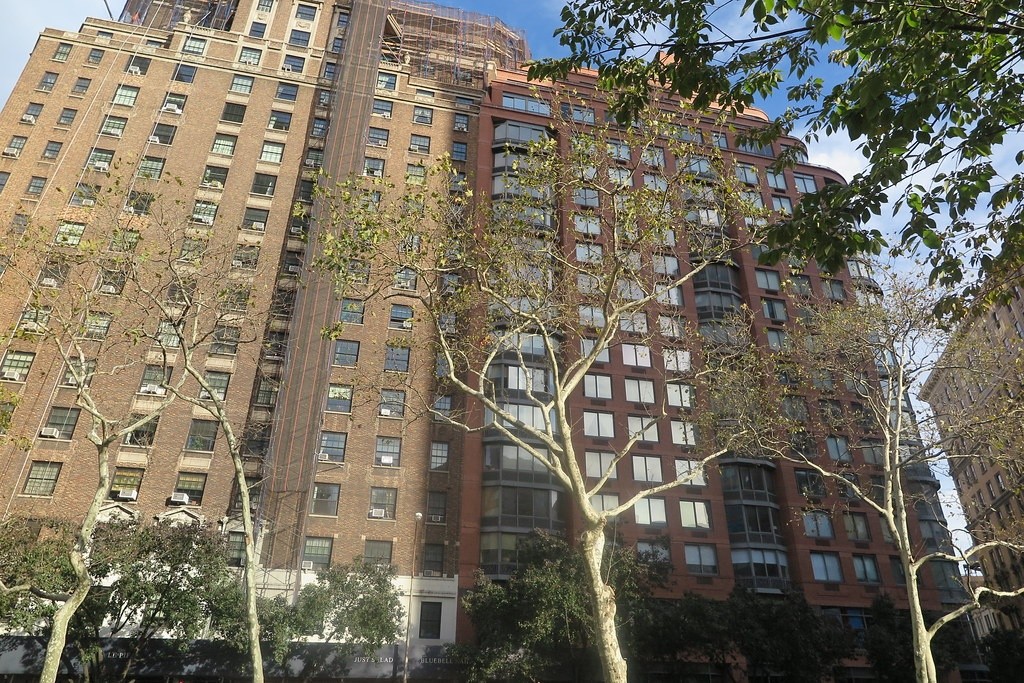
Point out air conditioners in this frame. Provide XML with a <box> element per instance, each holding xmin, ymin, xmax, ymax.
<box><xmin>424</xmin><ymin>569</ymin><xmax>434</xmax><ymax>577</ymax></box>
<box><xmin>170</xmin><ymin>491</ymin><xmax>190</xmax><ymax>504</ymax></box>
<box><xmin>0</xmin><ymin>65</ymin><xmax>443</xmax><ymax>525</ymax></box>
<box><xmin>118</xmin><ymin>488</ymin><xmax>138</xmax><ymax>501</ymax></box>
<box><xmin>301</xmin><ymin>560</ymin><xmax>314</xmax><ymax>570</ymax></box>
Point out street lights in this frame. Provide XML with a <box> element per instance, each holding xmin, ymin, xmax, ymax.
<box><xmin>403</xmin><ymin>512</ymin><xmax>424</xmax><ymax>683</ymax></box>
